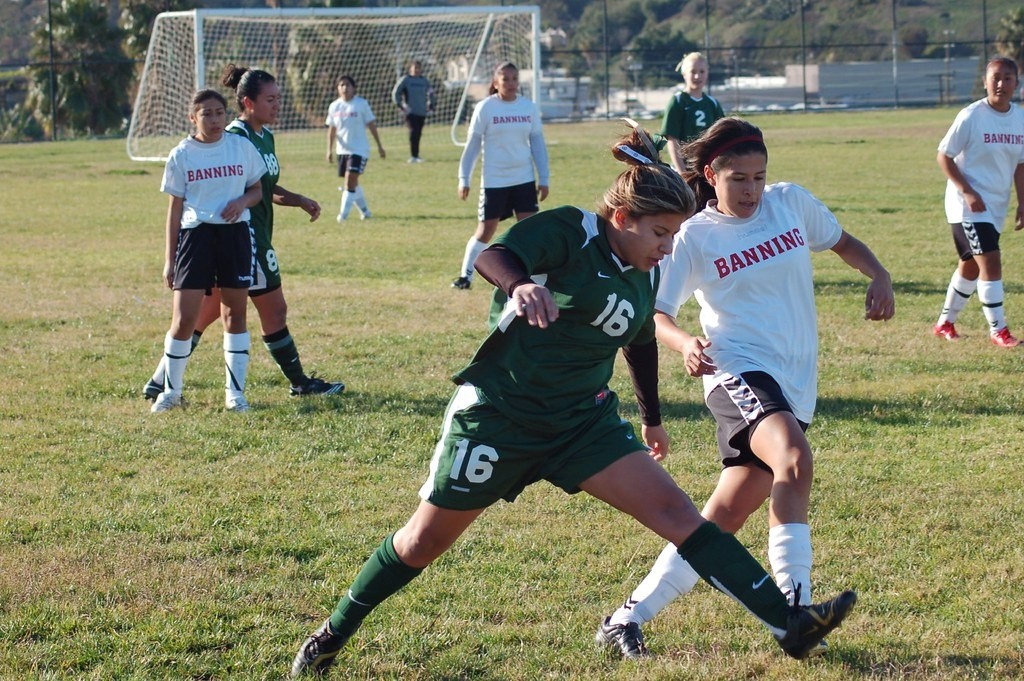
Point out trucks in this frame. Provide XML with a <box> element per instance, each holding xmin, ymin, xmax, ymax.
<box><xmin>518</xmin><ymin>78</ymin><xmax>597</xmax><ymax>121</ymax></box>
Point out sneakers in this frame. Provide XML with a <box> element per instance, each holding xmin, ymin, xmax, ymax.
<box><xmin>452</xmin><ymin>276</ymin><xmax>470</xmax><ymax>289</ymax></box>
<box><xmin>143</xmin><ymin>378</ymin><xmax>164</xmax><ymax>400</ymax></box>
<box><xmin>290</xmin><ymin>374</ymin><xmax>345</xmax><ymax>398</ymax></box>
<box><xmin>151</xmin><ymin>392</ymin><xmax>181</xmax><ymax>413</ymax></box>
<box><xmin>774</xmin><ymin>581</ymin><xmax>857</xmax><ymax>660</ymax></box>
<box><xmin>294</xmin><ymin>618</ymin><xmax>362</xmax><ymax>678</ymax></box>
<box><xmin>990</xmin><ymin>327</ymin><xmax>1023</xmax><ymax>347</ymax></box>
<box><xmin>226</xmin><ymin>390</ymin><xmax>248</xmax><ymax>410</ymax></box>
<box><xmin>596</xmin><ymin>616</ymin><xmax>649</xmax><ymax>658</ymax></box>
<box><xmin>933</xmin><ymin>320</ymin><xmax>961</xmax><ymax>340</ymax></box>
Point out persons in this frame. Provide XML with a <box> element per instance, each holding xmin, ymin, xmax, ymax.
<box><xmin>291</xmin><ymin>132</ymin><xmax>857</xmax><ymax>681</ymax></box>
<box><xmin>595</xmin><ymin>117</ymin><xmax>894</xmax><ymax>661</ymax></box>
<box><xmin>450</xmin><ymin>62</ymin><xmax>549</xmax><ymax>290</ymax></box>
<box><xmin>325</xmin><ymin>75</ymin><xmax>386</xmax><ymax>223</ymax></box>
<box><xmin>933</xmin><ymin>57</ymin><xmax>1024</xmax><ymax>348</ymax></box>
<box><xmin>392</xmin><ymin>60</ymin><xmax>437</xmax><ymax>164</ymax></box>
<box><xmin>661</xmin><ymin>51</ymin><xmax>726</xmax><ymax>213</ymax></box>
<box><xmin>142</xmin><ymin>65</ymin><xmax>344</xmax><ymax>413</ymax></box>
